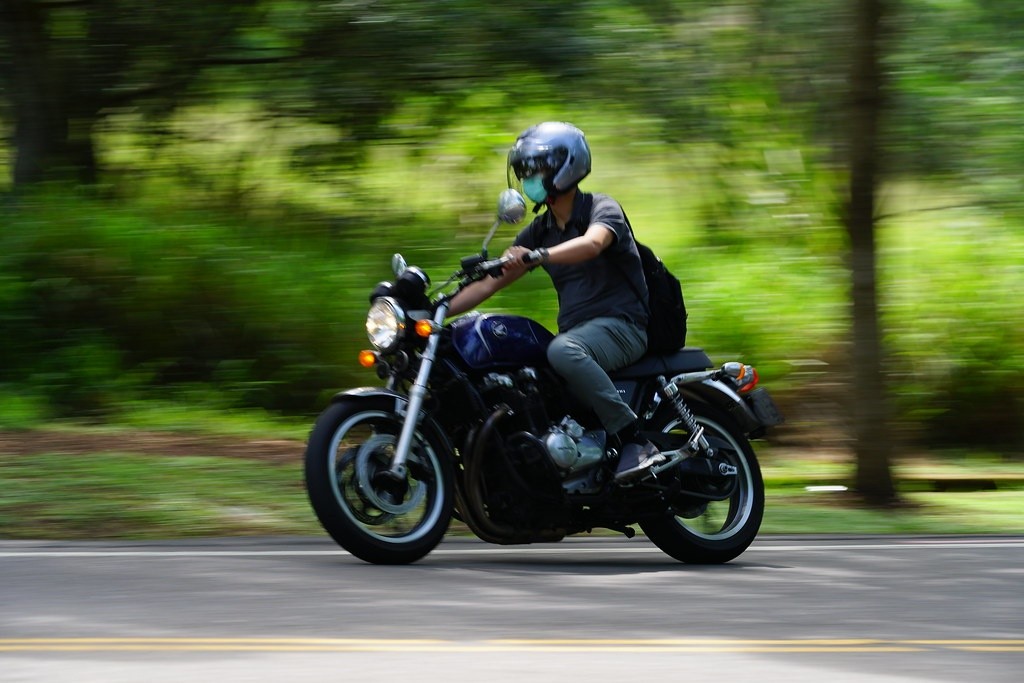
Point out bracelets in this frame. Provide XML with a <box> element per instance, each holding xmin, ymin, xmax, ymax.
<box><xmin>536</xmin><ymin>247</ymin><xmax>550</xmax><ymax>263</ymax></box>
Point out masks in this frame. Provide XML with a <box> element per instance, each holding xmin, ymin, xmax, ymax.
<box><xmin>522</xmin><ymin>172</ymin><xmax>549</xmax><ymax>205</ymax></box>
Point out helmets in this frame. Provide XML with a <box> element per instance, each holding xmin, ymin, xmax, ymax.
<box><xmin>506</xmin><ymin>121</ymin><xmax>593</xmax><ymax>214</ymax></box>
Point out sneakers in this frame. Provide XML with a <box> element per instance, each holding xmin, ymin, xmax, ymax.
<box><xmin>615</xmin><ymin>438</ymin><xmax>667</xmax><ymax>483</ymax></box>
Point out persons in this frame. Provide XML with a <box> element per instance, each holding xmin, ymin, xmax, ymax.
<box><xmin>433</xmin><ymin>120</ymin><xmax>667</xmax><ymax>479</ymax></box>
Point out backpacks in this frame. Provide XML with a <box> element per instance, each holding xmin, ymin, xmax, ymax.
<box><xmin>621</xmin><ymin>204</ymin><xmax>688</xmax><ymax>353</ymax></box>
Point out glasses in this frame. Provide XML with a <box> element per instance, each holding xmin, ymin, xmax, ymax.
<box><xmin>510</xmin><ymin>156</ymin><xmax>548</xmax><ymax>183</ymax></box>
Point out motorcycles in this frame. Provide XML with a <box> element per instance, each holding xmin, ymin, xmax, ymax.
<box><xmin>303</xmin><ymin>190</ymin><xmax>781</xmax><ymax>567</ymax></box>
